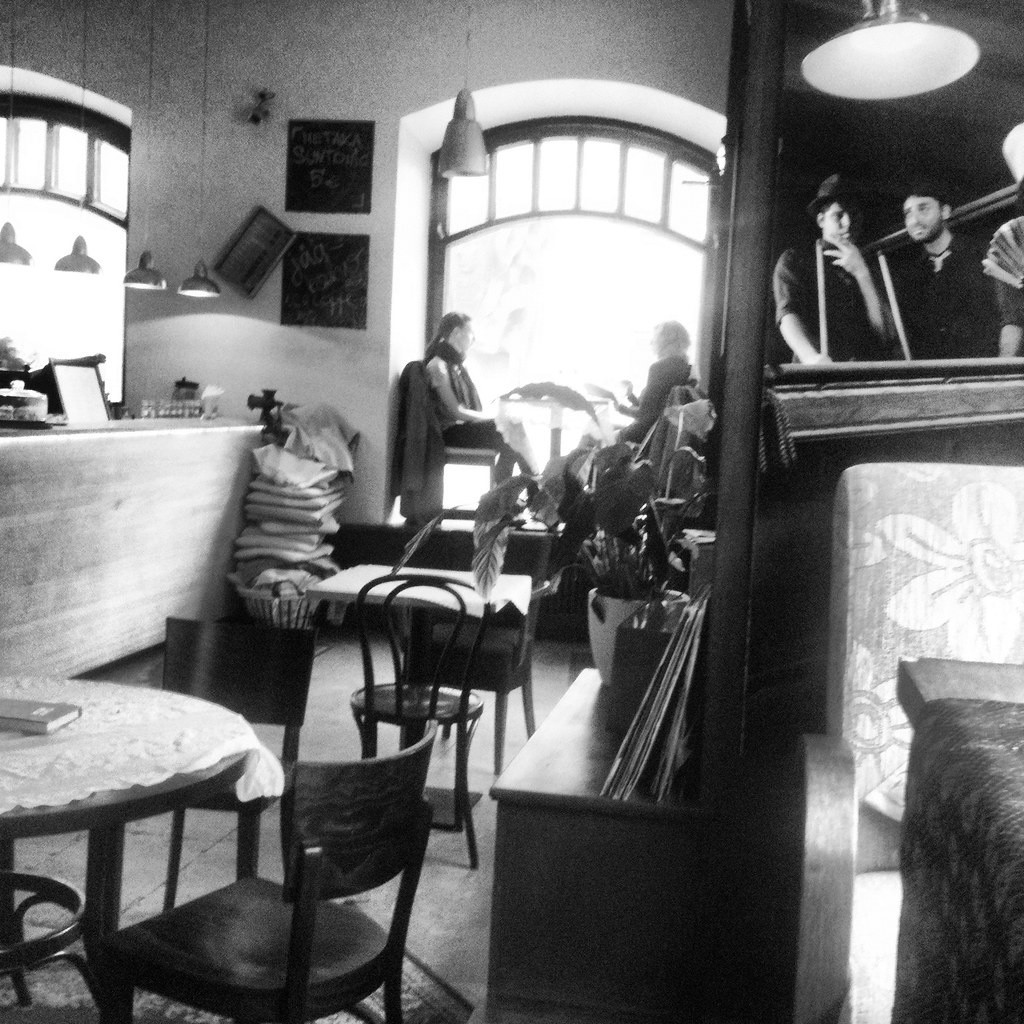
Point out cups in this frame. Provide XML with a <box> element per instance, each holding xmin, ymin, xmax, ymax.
<box><xmin>0</xmin><ymin>380</ymin><xmax>48</xmax><ymax>421</ymax></box>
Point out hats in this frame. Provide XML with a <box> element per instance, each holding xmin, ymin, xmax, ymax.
<box><xmin>807</xmin><ymin>175</ymin><xmax>864</xmax><ymax>219</ymax></box>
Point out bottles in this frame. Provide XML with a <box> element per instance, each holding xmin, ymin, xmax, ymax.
<box><xmin>141</xmin><ymin>399</ymin><xmax>201</xmax><ymax>416</ymax></box>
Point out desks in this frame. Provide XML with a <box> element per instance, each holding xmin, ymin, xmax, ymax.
<box><xmin>892</xmin><ymin>655</ymin><xmax>1024</xmax><ymax>1024</ymax></box>
<box><xmin>0</xmin><ymin>675</ymin><xmax>263</xmax><ymax>1024</ymax></box>
<box><xmin>497</xmin><ymin>393</ymin><xmax>612</xmax><ymax>532</ymax></box>
<box><xmin>305</xmin><ymin>560</ymin><xmax>537</xmax><ymax>827</ymax></box>
<box><xmin>487</xmin><ymin>664</ymin><xmax>714</xmax><ymax>1013</ymax></box>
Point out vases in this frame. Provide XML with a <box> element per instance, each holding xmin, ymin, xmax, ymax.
<box><xmin>592</xmin><ymin>585</ymin><xmax>700</xmax><ymax>682</ymax></box>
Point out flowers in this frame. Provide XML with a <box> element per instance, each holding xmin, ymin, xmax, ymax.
<box><xmin>583</xmin><ymin>484</ymin><xmax>709</xmax><ymax>593</ymax></box>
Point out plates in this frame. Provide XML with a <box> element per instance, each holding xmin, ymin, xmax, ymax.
<box><xmin>0</xmin><ymin>420</ymin><xmax>50</xmax><ymax>429</ymax></box>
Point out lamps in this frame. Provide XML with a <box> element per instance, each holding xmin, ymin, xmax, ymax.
<box><xmin>57</xmin><ymin>0</ymin><xmax>104</xmax><ymax>274</ymax></box>
<box><xmin>177</xmin><ymin>0</ymin><xmax>222</xmax><ymax>297</ymax></box>
<box><xmin>436</xmin><ymin>0</ymin><xmax>492</xmax><ymax>177</ymax></box>
<box><xmin>0</xmin><ymin>0</ymin><xmax>34</xmax><ymax>266</ymax></box>
<box><xmin>799</xmin><ymin>0</ymin><xmax>980</xmax><ymax>97</ymax></box>
<box><xmin>123</xmin><ymin>0</ymin><xmax>171</xmax><ymax>291</ymax></box>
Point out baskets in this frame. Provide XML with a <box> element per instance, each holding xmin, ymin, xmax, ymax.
<box><xmin>237</xmin><ymin>581</ymin><xmax>318</xmax><ymax>630</ymax></box>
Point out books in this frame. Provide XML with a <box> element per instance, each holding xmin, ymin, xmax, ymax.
<box><xmin>598</xmin><ymin>583</ymin><xmax>713</xmax><ymax>799</ymax></box>
<box><xmin>0</xmin><ymin>697</ymin><xmax>83</xmax><ymax>735</ymax></box>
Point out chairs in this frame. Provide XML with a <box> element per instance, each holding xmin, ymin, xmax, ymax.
<box><xmin>398</xmin><ymin>361</ymin><xmax>504</xmax><ymax>525</ymax></box>
<box><xmin>117</xmin><ymin>729</ymin><xmax>448</xmax><ymax>1024</ymax></box>
<box><xmin>160</xmin><ymin>615</ymin><xmax>320</xmax><ymax>909</ymax></box>
<box><xmin>351</xmin><ymin>573</ymin><xmax>493</xmax><ymax>863</ymax></box>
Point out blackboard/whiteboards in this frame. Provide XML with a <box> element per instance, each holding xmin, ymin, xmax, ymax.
<box><xmin>281</xmin><ymin>231</ymin><xmax>370</xmax><ymax>330</ymax></box>
<box><xmin>285</xmin><ymin>119</ymin><xmax>376</xmax><ymax>215</ymax></box>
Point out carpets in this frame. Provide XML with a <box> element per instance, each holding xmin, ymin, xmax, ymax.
<box><xmin>0</xmin><ymin>897</ymin><xmax>474</xmax><ymax>1024</ymax></box>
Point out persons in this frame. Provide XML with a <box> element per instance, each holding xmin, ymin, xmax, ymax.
<box><xmin>424</xmin><ymin>311</ymin><xmax>542</xmax><ymax>506</ymax></box>
<box><xmin>880</xmin><ymin>179</ymin><xmax>1024</xmax><ymax>357</ymax></box>
<box><xmin>576</xmin><ymin>320</ymin><xmax>697</xmax><ymax>448</ymax></box>
<box><xmin>773</xmin><ymin>173</ymin><xmax>895</xmax><ymax>364</ymax></box>
